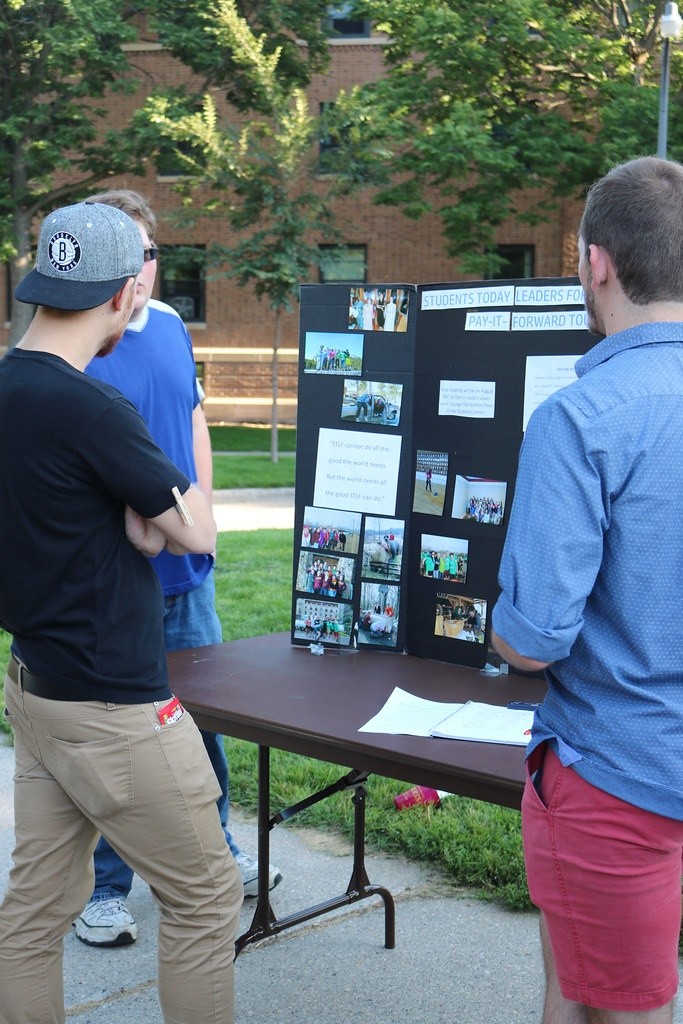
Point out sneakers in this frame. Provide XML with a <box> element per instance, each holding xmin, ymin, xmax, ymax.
<box><xmin>234</xmin><ymin>853</ymin><xmax>283</xmax><ymax>898</ymax></box>
<box><xmin>72</xmin><ymin>898</ymin><xmax>137</xmax><ymax>947</ymax></box>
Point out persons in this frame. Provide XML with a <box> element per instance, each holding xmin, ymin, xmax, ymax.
<box><xmin>305</xmin><ymin>559</ymin><xmax>347</xmax><ymax>598</ymax></box>
<box><xmin>468</xmin><ymin>495</ymin><xmax>503</xmax><ymax>525</ymax></box>
<box><xmin>2</xmin><ymin>203</ymin><xmax>245</xmax><ymax>1023</ymax></box>
<box><xmin>304</xmin><ymin>614</ymin><xmax>340</xmax><ymax>643</ymax></box>
<box><xmin>490</xmin><ymin>156</ymin><xmax>683</xmax><ymax>1024</ymax></box>
<box><xmin>420</xmin><ymin>550</ymin><xmax>465</xmax><ymax>581</ymax></box>
<box><xmin>316</xmin><ymin>347</ymin><xmax>352</xmax><ymax>371</ymax></box>
<box><xmin>453</xmin><ymin>605</ymin><xmax>482</xmax><ymax>631</ymax></box>
<box><xmin>355</xmin><ymin>392</ymin><xmax>384</xmax><ymax>422</ymax></box>
<box><xmin>71</xmin><ymin>186</ymin><xmax>284</xmax><ymax>951</ymax></box>
<box><xmin>302</xmin><ymin>522</ymin><xmax>346</xmax><ymax>552</ymax></box>
<box><xmin>425</xmin><ymin>469</ymin><xmax>433</xmax><ymax>491</ymax></box>
<box><xmin>360</xmin><ymin>601</ymin><xmax>395</xmax><ymax>634</ymax></box>
<box><xmin>348</xmin><ymin>294</ymin><xmax>407</xmax><ymax>332</ymax></box>
<box><xmin>384</xmin><ymin>529</ymin><xmax>395</xmax><ymax>542</ymax></box>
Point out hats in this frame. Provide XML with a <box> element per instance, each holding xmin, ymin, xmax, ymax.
<box><xmin>15</xmin><ymin>201</ymin><xmax>144</xmax><ymax>311</ymax></box>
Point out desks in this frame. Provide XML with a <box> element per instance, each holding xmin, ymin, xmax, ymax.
<box><xmin>167</xmin><ymin>631</ymin><xmax>547</xmax><ymax>965</ymax></box>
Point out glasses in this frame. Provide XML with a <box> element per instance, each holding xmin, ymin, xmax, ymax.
<box><xmin>144</xmin><ymin>241</ymin><xmax>159</xmax><ymax>262</ymax></box>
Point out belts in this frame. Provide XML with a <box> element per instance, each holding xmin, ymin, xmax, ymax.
<box><xmin>6</xmin><ymin>653</ymin><xmax>97</xmax><ymax>701</ymax></box>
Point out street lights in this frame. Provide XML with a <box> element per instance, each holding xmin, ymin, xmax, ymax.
<box><xmin>656</xmin><ymin>6</ymin><xmax>683</xmax><ymax>161</ymax></box>
